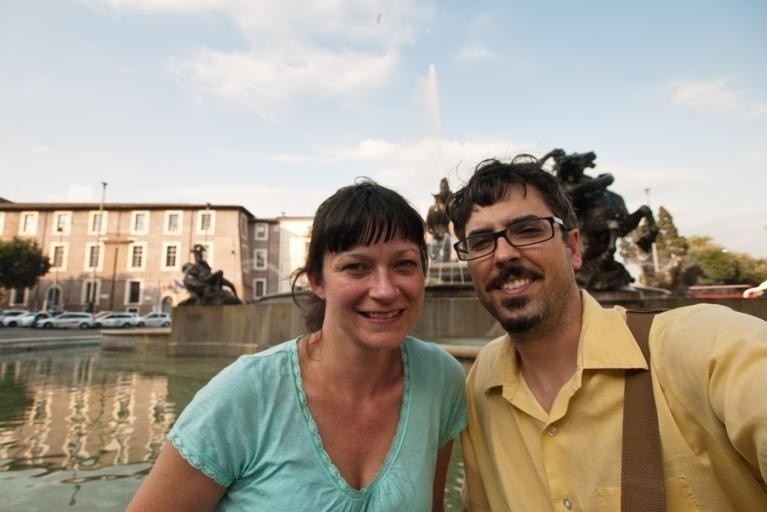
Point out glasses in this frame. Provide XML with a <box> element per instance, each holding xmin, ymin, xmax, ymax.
<box><xmin>454</xmin><ymin>217</ymin><xmax>566</xmax><ymax>262</ymax></box>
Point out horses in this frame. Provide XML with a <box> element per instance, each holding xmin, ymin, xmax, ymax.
<box><xmin>552</xmin><ymin>150</ymin><xmax>659</xmax><ymax>269</ymax></box>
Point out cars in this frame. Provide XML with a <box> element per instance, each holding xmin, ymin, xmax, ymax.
<box><xmin>37</xmin><ymin>312</ymin><xmax>93</xmax><ymax>330</ymax></box>
<box><xmin>0</xmin><ymin>306</ymin><xmax>58</xmax><ymax>329</ymax></box>
<box><xmin>95</xmin><ymin>313</ymin><xmax>140</xmax><ymax>328</ymax></box>
<box><xmin>92</xmin><ymin>311</ymin><xmax>106</xmax><ymax>319</ymax></box>
<box><xmin>139</xmin><ymin>312</ymin><xmax>170</xmax><ymax>327</ymax></box>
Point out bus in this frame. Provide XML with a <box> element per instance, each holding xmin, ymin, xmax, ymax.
<box><xmin>685</xmin><ymin>283</ymin><xmax>754</xmax><ymax>301</ymax></box>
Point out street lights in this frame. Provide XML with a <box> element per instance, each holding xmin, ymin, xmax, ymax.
<box><xmin>88</xmin><ymin>181</ymin><xmax>108</xmax><ymax>313</ymax></box>
<box><xmin>642</xmin><ymin>188</ymin><xmax>662</xmax><ymax>275</ymax></box>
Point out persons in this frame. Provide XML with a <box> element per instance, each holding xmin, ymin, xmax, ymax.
<box><xmin>124</xmin><ymin>180</ymin><xmax>468</xmax><ymax>512</ymax></box>
<box><xmin>446</xmin><ymin>153</ymin><xmax>767</xmax><ymax>511</ymax></box>
<box><xmin>188</xmin><ymin>243</ymin><xmax>237</xmax><ymax>298</ymax></box>
